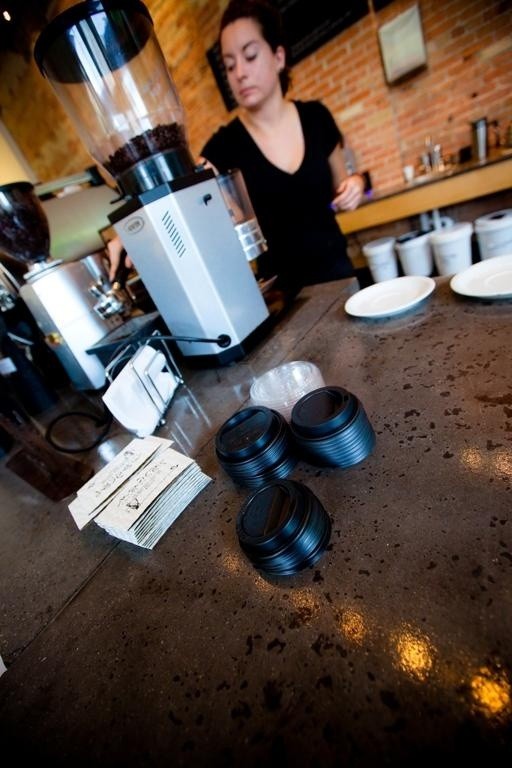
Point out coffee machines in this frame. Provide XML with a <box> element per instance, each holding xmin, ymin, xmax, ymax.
<box><xmin>0</xmin><ymin>180</ymin><xmax>179</xmax><ymax>396</ymax></box>
<box><xmin>32</xmin><ymin>0</ymin><xmax>306</xmax><ymax>362</ymax></box>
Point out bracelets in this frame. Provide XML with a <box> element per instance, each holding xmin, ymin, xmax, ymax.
<box><xmin>352</xmin><ymin>167</ymin><xmax>368</xmax><ymax>192</ymax></box>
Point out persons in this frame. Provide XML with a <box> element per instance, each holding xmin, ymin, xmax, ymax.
<box><xmin>105</xmin><ymin>1</ymin><xmax>371</xmax><ymax>283</ymax></box>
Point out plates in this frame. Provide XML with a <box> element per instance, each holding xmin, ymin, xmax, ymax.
<box><xmin>450</xmin><ymin>254</ymin><xmax>511</xmax><ymax>301</ymax></box>
<box><xmin>344</xmin><ymin>275</ymin><xmax>437</xmax><ymax>321</ymax></box>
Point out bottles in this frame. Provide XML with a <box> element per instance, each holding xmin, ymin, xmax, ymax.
<box><xmin>416</xmin><ymin>135</ymin><xmax>452</xmax><ymax>177</ymax></box>
<box><xmin>471</xmin><ymin>118</ymin><xmax>491</xmax><ymax>161</ymax></box>
<box><xmin>484</xmin><ymin>122</ymin><xmax>499</xmax><ymax>149</ymax></box>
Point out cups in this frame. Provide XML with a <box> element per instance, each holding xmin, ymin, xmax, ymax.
<box><xmin>457</xmin><ymin>145</ymin><xmax>473</xmax><ymax>163</ymax></box>
<box><xmin>401</xmin><ymin>166</ymin><xmax>415</xmax><ymax>183</ymax></box>
